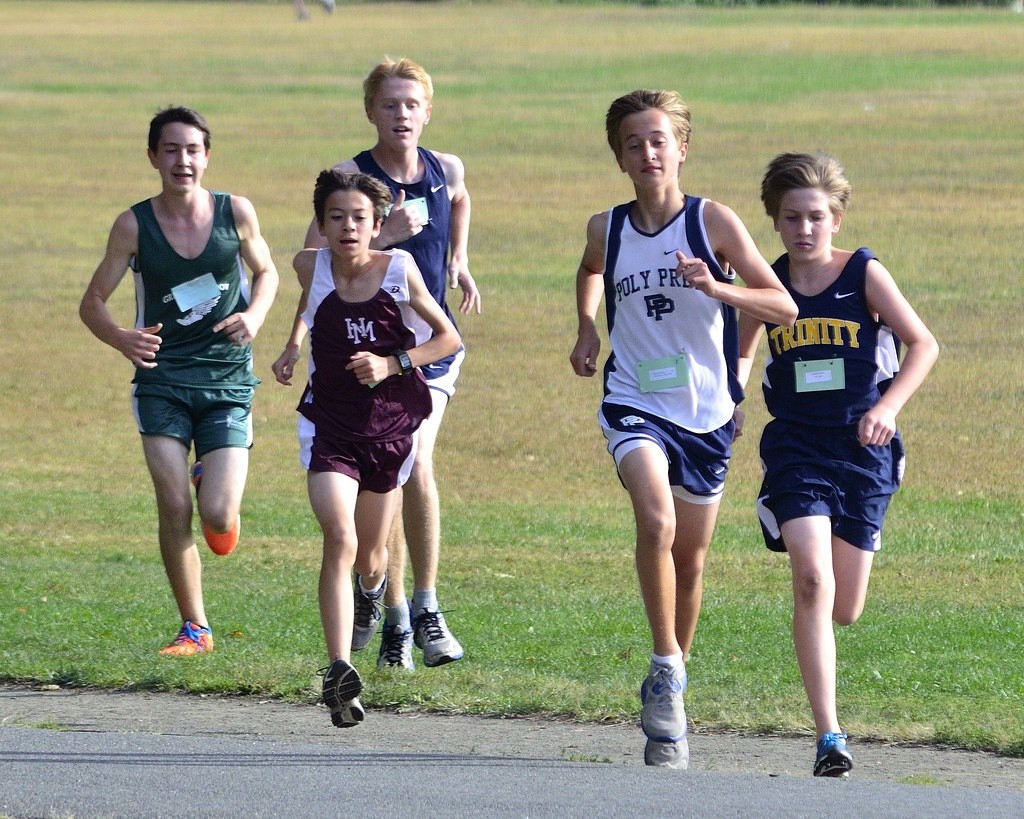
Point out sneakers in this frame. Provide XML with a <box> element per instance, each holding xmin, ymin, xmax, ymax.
<box><xmin>644</xmin><ymin>738</ymin><xmax>690</xmax><ymax>770</ymax></box>
<box><xmin>351</xmin><ymin>572</ymin><xmax>387</xmax><ymax>651</ymax></box>
<box><xmin>413</xmin><ymin>607</ymin><xmax>465</xmax><ymax>667</ymax></box>
<box><xmin>641</xmin><ymin>658</ymin><xmax>688</xmax><ymax>742</ymax></box>
<box><xmin>316</xmin><ymin>653</ymin><xmax>364</xmax><ymax>728</ymax></box>
<box><xmin>190</xmin><ymin>462</ymin><xmax>241</xmax><ymax>555</ymax></box>
<box><xmin>814</xmin><ymin>731</ymin><xmax>853</xmax><ymax>777</ymax></box>
<box><xmin>158</xmin><ymin>620</ymin><xmax>213</xmax><ymax>657</ymax></box>
<box><xmin>377</xmin><ymin>600</ymin><xmax>415</xmax><ymax>672</ymax></box>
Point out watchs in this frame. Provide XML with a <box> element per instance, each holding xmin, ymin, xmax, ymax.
<box><xmin>391</xmin><ymin>347</ymin><xmax>413</xmax><ymax>374</ymax></box>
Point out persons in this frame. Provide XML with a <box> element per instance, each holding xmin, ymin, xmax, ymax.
<box><xmin>732</xmin><ymin>152</ymin><xmax>938</xmax><ymax>777</ymax></box>
<box><xmin>271</xmin><ymin>169</ymin><xmax>462</xmax><ymax>728</ymax></box>
<box><xmin>302</xmin><ymin>55</ymin><xmax>480</xmax><ymax>667</ymax></box>
<box><xmin>78</xmin><ymin>107</ymin><xmax>279</xmax><ymax>656</ymax></box>
<box><xmin>568</xmin><ymin>89</ymin><xmax>799</xmax><ymax>768</ymax></box>
